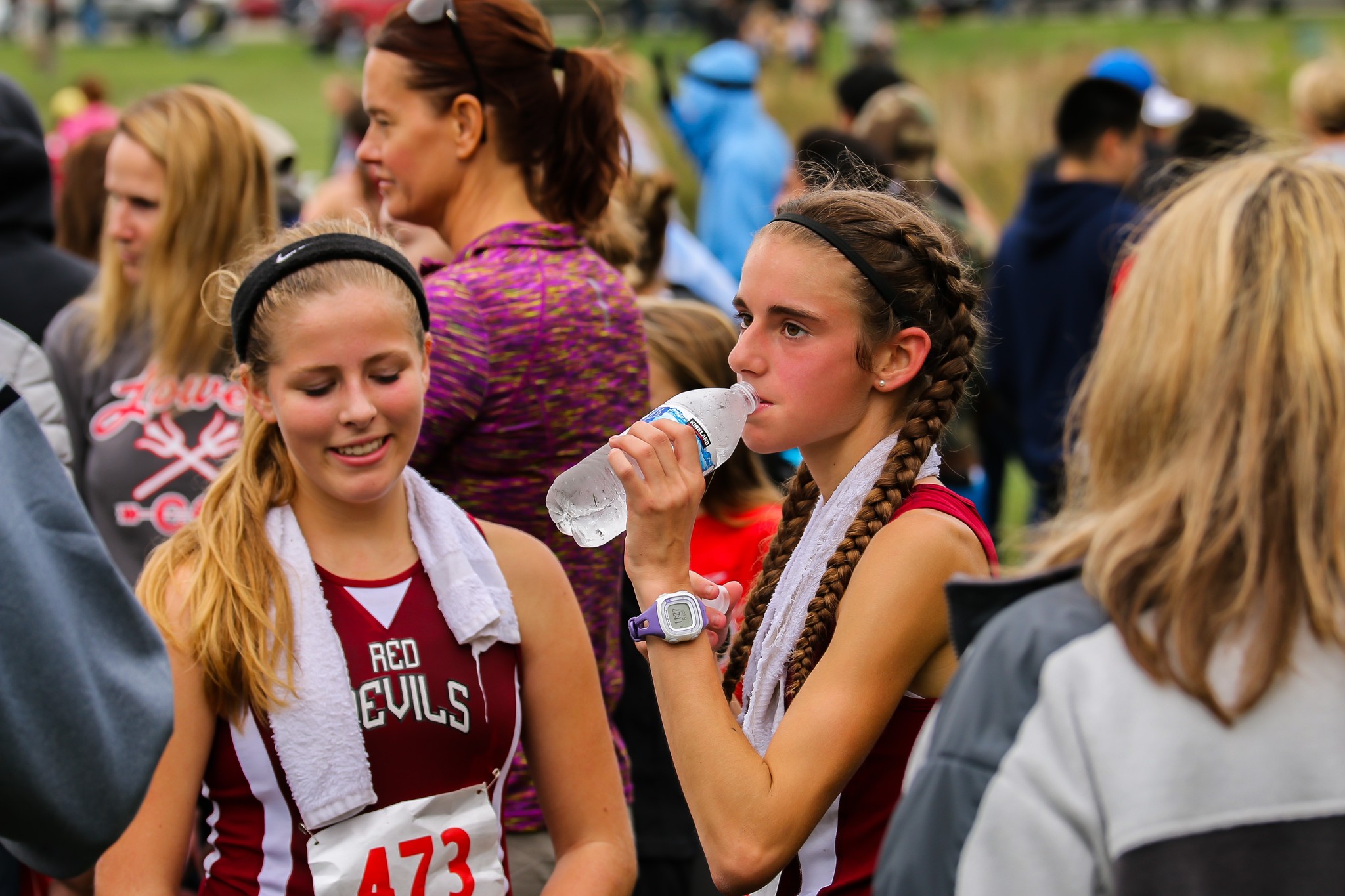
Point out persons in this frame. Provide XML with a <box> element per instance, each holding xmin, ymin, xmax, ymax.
<box><xmin>0</xmin><ymin>1</ymin><xmax>1345</xmax><ymax>896</ymax></box>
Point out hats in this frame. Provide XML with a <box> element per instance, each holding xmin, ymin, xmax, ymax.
<box><xmin>1091</xmin><ymin>51</ymin><xmax>1191</xmax><ymax>127</ymax></box>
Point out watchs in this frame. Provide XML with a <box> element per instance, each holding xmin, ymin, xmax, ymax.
<box><xmin>627</xmin><ymin>591</ymin><xmax>710</xmax><ymax>644</ymax></box>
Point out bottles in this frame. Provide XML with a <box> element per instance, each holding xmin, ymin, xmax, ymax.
<box><xmin>546</xmin><ymin>382</ymin><xmax>759</xmax><ymax>548</ymax></box>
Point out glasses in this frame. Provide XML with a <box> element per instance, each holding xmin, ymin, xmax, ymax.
<box><xmin>408</xmin><ymin>1</ymin><xmax>480</xmax><ymax>79</ymax></box>
